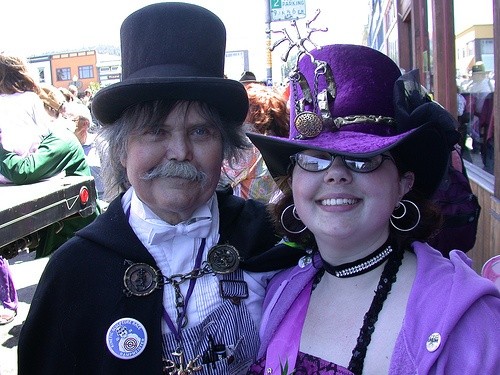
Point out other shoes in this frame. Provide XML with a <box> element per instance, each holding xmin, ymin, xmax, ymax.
<box><xmin>0</xmin><ymin>310</ymin><xmax>16</xmax><ymax>325</ymax></box>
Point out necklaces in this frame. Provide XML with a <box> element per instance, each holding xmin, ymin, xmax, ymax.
<box><xmin>320</xmin><ymin>235</ymin><xmax>396</xmax><ymax>278</ymax></box>
<box><xmin>123</xmin><ymin>204</ymin><xmax>205</xmax><ymax>375</ymax></box>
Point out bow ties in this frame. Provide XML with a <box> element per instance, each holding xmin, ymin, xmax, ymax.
<box><xmin>147</xmin><ymin>217</ymin><xmax>212</xmax><ymax>245</ymax></box>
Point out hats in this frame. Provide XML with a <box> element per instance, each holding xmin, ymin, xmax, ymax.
<box><xmin>470</xmin><ymin>61</ymin><xmax>490</xmax><ymax>74</ymax></box>
<box><xmin>91</xmin><ymin>2</ymin><xmax>249</xmax><ymax>126</ymax></box>
<box><xmin>237</xmin><ymin>71</ymin><xmax>267</xmax><ymax>86</ymax></box>
<box><xmin>245</xmin><ymin>44</ymin><xmax>458</xmax><ymax>199</ymax></box>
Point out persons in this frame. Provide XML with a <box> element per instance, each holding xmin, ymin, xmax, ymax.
<box><xmin>0</xmin><ymin>255</ymin><xmax>18</xmax><ymax>324</ymax></box>
<box><xmin>0</xmin><ymin>53</ymin><xmax>296</xmax><ymax>250</ymax></box>
<box><xmin>422</xmin><ymin>149</ymin><xmax>480</xmax><ymax>259</ymax></box>
<box><xmin>249</xmin><ymin>117</ymin><xmax>500</xmax><ymax>375</ymax></box>
<box><xmin>18</xmin><ymin>60</ymin><xmax>306</xmax><ymax>375</ymax></box>
<box><xmin>455</xmin><ymin>58</ymin><xmax>495</xmax><ymax>176</ymax></box>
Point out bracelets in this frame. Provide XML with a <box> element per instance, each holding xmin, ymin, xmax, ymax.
<box><xmin>56</xmin><ymin>102</ymin><xmax>63</xmax><ymax>112</ymax></box>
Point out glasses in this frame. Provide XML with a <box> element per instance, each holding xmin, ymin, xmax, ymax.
<box><xmin>287</xmin><ymin>148</ymin><xmax>400</xmax><ymax>172</ymax></box>
